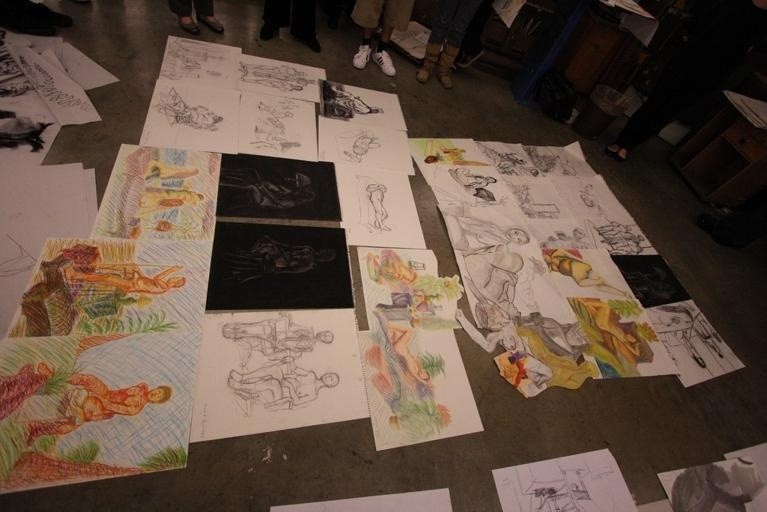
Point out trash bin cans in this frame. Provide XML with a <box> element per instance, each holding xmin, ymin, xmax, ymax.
<box><xmin>572</xmin><ymin>82</ymin><xmax>632</xmax><ymax>142</ymax></box>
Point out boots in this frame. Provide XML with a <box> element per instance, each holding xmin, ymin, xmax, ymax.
<box><xmin>413</xmin><ymin>39</ymin><xmax>442</xmax><ymax>85</ymax></box>
<box><xmin>434</xmin><ymin>43</ymin><xmax>460</xmax><ymax>90</ymax></box>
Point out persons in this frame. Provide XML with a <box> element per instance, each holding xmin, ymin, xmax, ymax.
<box><xmin>607</xmin><ymin>61</ymin><xmax>714</xmax><ymax>163</ymax></box>
<box><xmin>169</xmin><ymin>0</ymin><xmax>224</xmax><ymax>36</ymax></box>
<box><xmin>416</xmin><ymin>0</ymin><xmax>481</xmax><ymax>89</ymax></box>
<box><xmin>351</xmin><ymin>0</ymin><xmax>415</xmax><ymax>77</ymax></box>
<box><xmin>260</xmin><ymin>0</ymin><xmax>321</xmax><ymax>53</ymax></box>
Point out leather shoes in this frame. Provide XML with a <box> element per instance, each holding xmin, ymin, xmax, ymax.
<box><xmin>178</xmin><ymin>21</ymin><xmax>202</xmax><ymax>37</ymax></box>
<box><xmin>195</xmin><ymin>13</ymin><xmax>225</xmax><ymax>35</ymax></box>
<box><xmin>604</xmin><ymin>145</ymin><xmax>628</xmax><ymax>164</ymax></box>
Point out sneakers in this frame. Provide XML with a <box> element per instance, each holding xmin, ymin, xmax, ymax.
<box><xmin>326</xmin><ymin>13</ymin><xmax>339</xmax><ymax>31</ymax></box>
<box><xmin>350</xmin><ymin>43</ymin><xmax>372</xmax><ymax>71</ymax></box>
<box><xmin>308</xmin><ymin>39</ymin><xmax>323</xmax><ymax>54</ymax></box>
<box><xmin>371</xmin><ymin>46</ymin><xmax>396</xmax><ymax>78</ymax></box>
<box><xmin>259</xmin><ymin>22</ymin><xmax>280</xmax><ymax>41</ymax></box>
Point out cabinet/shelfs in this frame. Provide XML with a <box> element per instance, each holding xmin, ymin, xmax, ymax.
<box><xmin>670</xmin><ymin>103</ymin><xmax>767</xmax><ymax>207</ymax></box>
